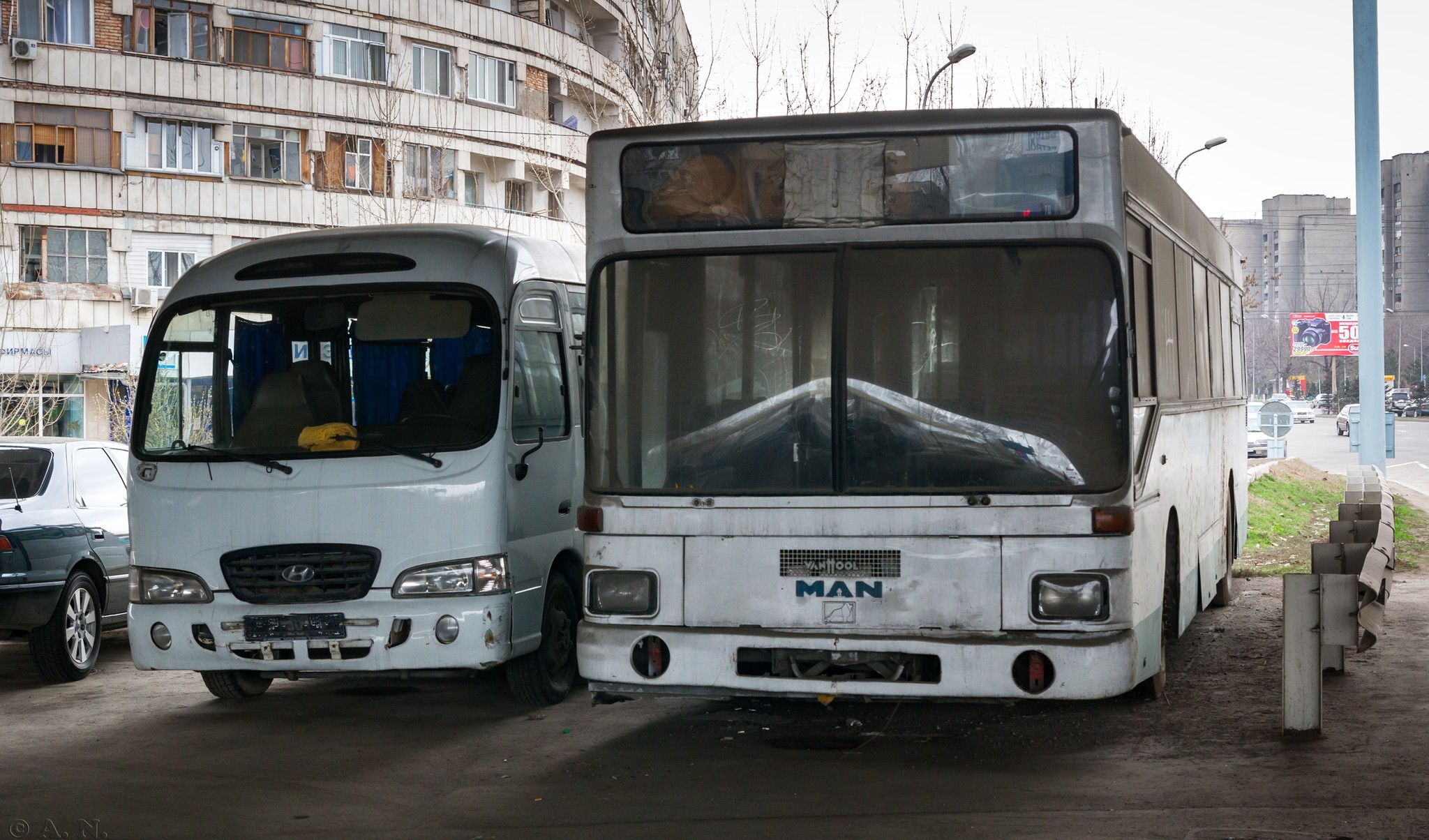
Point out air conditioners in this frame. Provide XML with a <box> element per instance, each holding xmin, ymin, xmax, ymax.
<box><xmin>9</xmin><ymin>37</ymin><xmax>37</xmax><ymax>60</ymax></box>
<box><xmin>661</xmin><ymin>54</ymin><xmax>669</xmax><ymax>67</ymax></box>
<box><xmin>131</xmin><ymin>286</ymin><xmax>158</xmax><ymax>308</ymax></box>
<box><xmin>1388</xmin><ymin>287</ymin><xmax>1393</xmax><ymax>291</ymax></box>
<box><xmin>689</xmin><ymin>95</ymin><xmax>695</xmax><ymax>106</ymax></box>
<box><xmin>683</xmin><ymin>109</ymin><xmax>689</xmax><ymax>118</ymax></box>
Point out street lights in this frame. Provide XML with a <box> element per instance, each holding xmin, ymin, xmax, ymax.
<box><xmin>1172</xmin><ymin>134</ymin><xmax>1228</xmax><ymax>186</ymax></box>
<box><xmin>1261</xmin><ymin>314</ymin><xmax>1280</xmax><ymax>393</ymax></box>
<box><xmin>1243</xmin><ymin>317</ymin><xmax>1255</xmax><ymax>397</ymax></box>
<box><xmin>923</xmin><ymin>44</ymin><xmax>975</xmax><ymax>111</ymax></box>
<box><xmin>1403</xmin><ymin>344</ymin><xmax>1415</xmax><ymax>372</ymax></box>
<box><xmin>1386</xmin><ymin>308</ymin><xmax>1401</xmax><ymax>388</ymax></box>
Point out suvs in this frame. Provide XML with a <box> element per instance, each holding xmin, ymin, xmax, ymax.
<box><xmin>1387</xmin><ymin>391</ymin><xmax>1411</xmax><ymax>417</ymax></box>
<box><xmin>1268</xmin><ymin>394</ymin><xmax>1287</xmax><ymax>403</ymax></box>
<box><xmin>1313</xmin><ymin>393</ymin><xmax>1333</xmax><ymax>408</ymax></box>
<box><xmin>1392</xmin><ymin>389</ymin><xmax>1413</xmax><ymax>397</ymax></box>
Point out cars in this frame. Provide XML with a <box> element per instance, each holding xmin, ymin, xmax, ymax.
<box><xmin>1403</xmin><ymin>398</ymin><xmax>1429</xmax><ymax>417</ymax></box>
<box><xmin>1247</xmin><ymin>402</ymin><xmax>1286</xmax><ymax>458</ymax></box>
<box><xmin>1280</xmin><ymin>401</ymin><xmax>1315</xmax><ymax>423</ymax></box>
<box><xmin>1</xmin><ymin>435</ymin><xmax>130</xmax><ymax>680</ymax></box>
<box><xmin>1283</xmin><ymin>396</ymin><xmax>1308</xmax><ymax>402</ymax></box>
<box><xmin>1336</xmin><ymin>403</ymin><xmax>1359</xmax><ymax>437</ymax></box>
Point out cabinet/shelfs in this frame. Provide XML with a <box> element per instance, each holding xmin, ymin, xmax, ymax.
<box><xmin>157</xmin><ymin>31</ymin><xmax>207</xmax><ymax>56</ymax></box>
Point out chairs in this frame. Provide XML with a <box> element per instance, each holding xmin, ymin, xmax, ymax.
<box><xmin>394</xmin><ymin>379</ymin><xmax>450</xmax><ymax>424</ymax></box>
<box><xmin>0</xmin><ymin>477</ymin><xmax>29</xmax><ymax>499</ymax></box>
<box><xmin>289</xmin><ymin>360</ymin><xmax>350</xmax><ymax>425</ymax></box>
<box><xmin>229</xmin><ymin>371</ymin><xmax>318</xmax><ymax>449</ymax></box>
<box><xmin>446</xmin><ymin>354</ymin><xmax>493</xmax><ymax>432</ymax></box>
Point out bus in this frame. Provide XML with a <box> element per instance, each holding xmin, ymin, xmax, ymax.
<box><xmin>573</xmin><ymin>124</ymin><xmax>1247</xmax><ymax>708</ymax></box>
<box><xmin>122</xmin><ymin>220</ymin><xmax>595</xmax><ymax>700</ymax></box>
